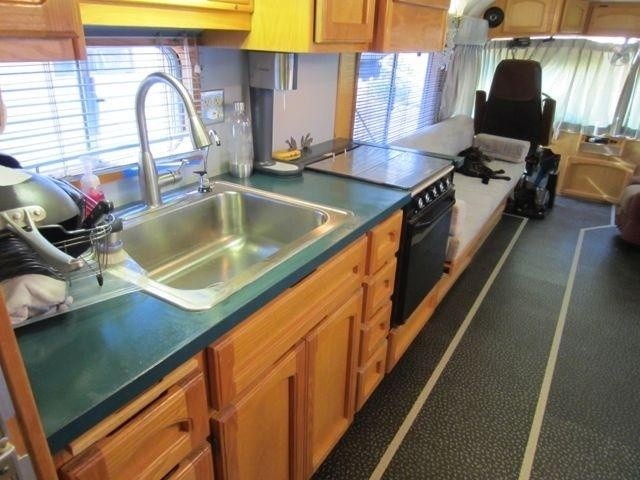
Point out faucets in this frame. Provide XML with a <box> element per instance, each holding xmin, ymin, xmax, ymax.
<box><xmin>134</xmin><ymin>71</ymin><xmax>212</xmax><ymax>208</ymax></box>
<box><xmin>193</xmin><ymin>130</ymin><xmax>221</xmax><ymax>192</ymax></box>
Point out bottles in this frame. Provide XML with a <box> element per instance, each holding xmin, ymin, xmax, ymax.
<box><xmin>226</xmin><ymin>101</ymin><xmax>254</xmax><ymax>178</ymax></box>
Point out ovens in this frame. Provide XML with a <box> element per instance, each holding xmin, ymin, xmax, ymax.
<box><xmin>391</xmin><ymin>189</ymin><xmax>456</xmax><ymax>326</ymax></box>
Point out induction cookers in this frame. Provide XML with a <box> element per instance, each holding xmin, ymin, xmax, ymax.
<box><xmin>305</xmin><ymin>144</ymin><xmax>456</xmax><ymax>220</ymax></box>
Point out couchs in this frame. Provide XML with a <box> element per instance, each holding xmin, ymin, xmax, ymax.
<box><xmin>388</xmin><ymin>114</ymin><xmax>532</xmax><ymax>283</ymax></box>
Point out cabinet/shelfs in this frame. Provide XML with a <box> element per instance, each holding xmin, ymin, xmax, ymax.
<box><xmin>0</xmin><ymin>0</ymin><xmax>89</xmax><ymax>63</ymax></box>
<box><xmin>56</xmin><ymin>354</ymin><xmax>222</xmax><ymax>480</ymax></box>
<box><xmin>75</xmin><ymin>1</ymin><xmax>255</xmax><ymax>41</ymax></box>
<box><xmin>370</xmin><ymin>0</ymin><xmax>451</xmax><ymax>53</ymax></box>
<box><xmin>355</xmin><ymin>210</ymin><xmax>404</xmax><ymax>415</ymax></box>
<box><xmin>480</xmin><ymin>0</ymin><xmax>640</xmax><ymax>37</ymax></box>
<box><xmin>188</xmin><ymin>0</ymin><xmax>378</xmax><ymax>57</ymax></box>
<box><xmin>206</xmin><ymin>231</ymin><xmax>369</xmax><ymax>480</ymax></box>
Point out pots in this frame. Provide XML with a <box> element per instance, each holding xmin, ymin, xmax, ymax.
<box><xmin>0</xmin><ymin>168</ymin><xmax>84</xmax><ymax>274</ymax></box>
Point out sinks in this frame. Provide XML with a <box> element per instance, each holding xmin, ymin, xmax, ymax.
<box><xmin>119</xmin><ymin>187</ymin><xmax>336</xmax><ymax>296</ymax></box>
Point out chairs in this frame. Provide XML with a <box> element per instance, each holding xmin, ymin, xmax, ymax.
<box><xmin>474</xmin><ymin>59</ymin><xmax>556</xmax><ymax>175</ymax></box>
